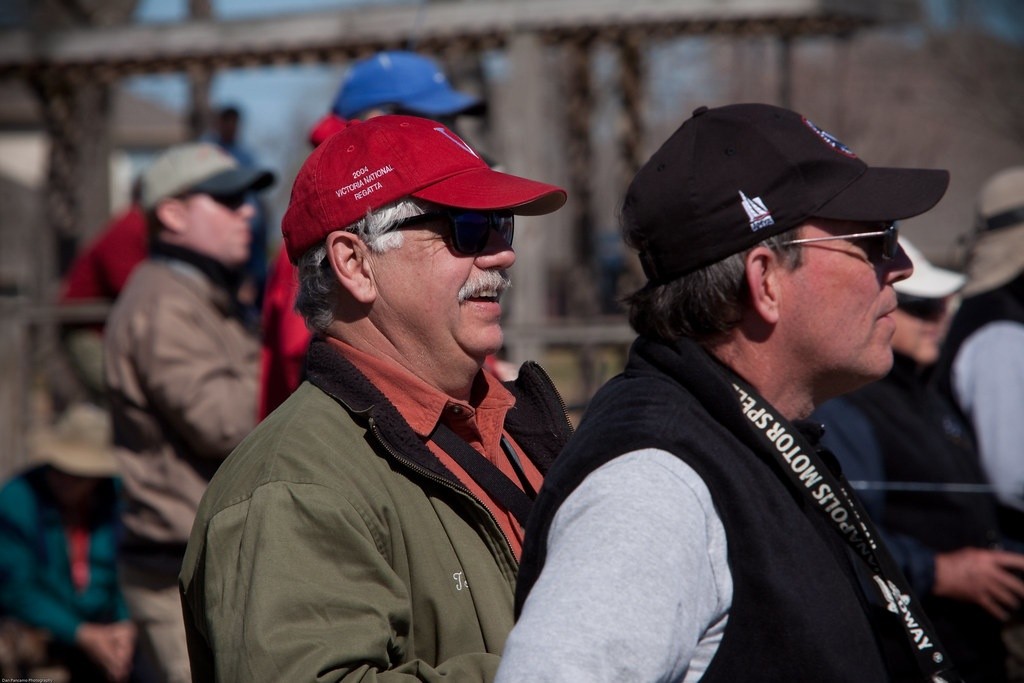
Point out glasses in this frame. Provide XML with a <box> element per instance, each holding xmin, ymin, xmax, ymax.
<box><xmin>766</xmin><ymin>221</ymin><xmax>900</xmax><ymax>264</ymax></box>
<box><xmin>317</xmin><ymin>207</ymin><xmax>514</xmax><ymax>275</ymax></box>
<box><xmin>177</xmin><ymin>191</ymin><xmax>251</xmax><ymax>210</ymax></box>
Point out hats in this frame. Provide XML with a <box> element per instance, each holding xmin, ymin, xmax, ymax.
<box><xmin>332</xmin><ymin>51</ymin><xmax>485</xmax><ymax>116</ymax></box>
<box><xmin>26</xmin><ymin>403</ymin><xmax>120</xmax><ymax>479</ymax></box>
<box><xmin>310</xmin><ymin>110</ymin><xmax>351</xmax><ymax>145</ymax></box>
<box><xmin>282</xmin><ymin>116</ymin><xmax>565</xmax><ymax>263</ymax></box>
<box><xmin>889</xmin><ymin>232</ymin><xmax>966</xmax><ymax>297</ymax></box>
<box><xmin>623</xmin><ymin>104</ymin><xmax>951</xmax><ymax>287</ymax></box>
<box><xmin>959</xmin><ymin>167</ymin><xmax>1024</xmax><ymax>298</ymax></box>
<box><xmin>141</xmin><ymin>144</ymin><xmax>275</xmax><ymax>215</ymax></box>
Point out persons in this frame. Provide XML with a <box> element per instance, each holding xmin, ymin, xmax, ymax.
<box><xmin>509</xmin><ymin>98</ymin><xmax>956</xmax><ymax>681</ymax></box>
<box><xmin>55</xmin><ymin>122</ymin><xmax>310</xmax><ymax>682</ymax></box>
<box><xmin>179</xmin><ymin>113</ymin><xmax>546</xmax><ymax>683</ymax></box>
<box><xmin>0</xmin><ymin>399</ymin><xmax>168</xmax><ymax>682</ymax></box>
<box><xmin>814</xmin><ymin>163</ymin><xmax>1023</xmax><ymax>683</ymax></box>
